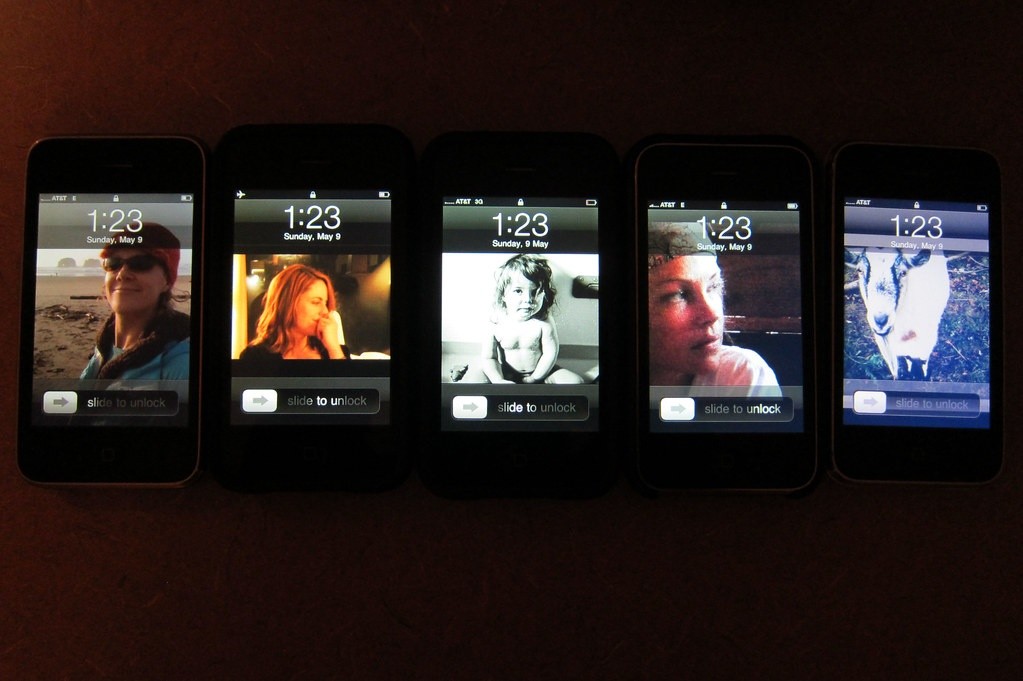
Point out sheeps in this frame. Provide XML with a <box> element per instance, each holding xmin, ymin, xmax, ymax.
<box><xmin>845</xmin><ymin>246</ymin><xmax>950</xmax><ymax>380</ymax></box>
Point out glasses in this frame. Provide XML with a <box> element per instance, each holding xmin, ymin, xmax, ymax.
<box><xmin>101</xmin><ymin>253</ymin><xmax>166</xmax><ymax>272</ymax></box>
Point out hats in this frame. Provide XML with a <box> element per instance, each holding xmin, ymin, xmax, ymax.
<box><xmin>99</xmin><ymin>221</ymin><xmax>180</xmax><ymax>285</ymax></box>
<box><xmin>647</xmin><ymin>222</ymin><xmax>717</xmax><ymax>270</ymax></box>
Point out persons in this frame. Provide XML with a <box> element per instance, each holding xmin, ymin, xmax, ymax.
<box><xmin>77</xmin><ymin>222</ymin><xmax>190</xmax><ymax>402</ymax></box>
<box><xmin>238</xmin><ymin>264</ymin><xmax>367</xmax><ymax>378</ymax></box>
<box><xmin>649</xmin><ymin>221</ymin><xmax>784</xmax><ymax>429</ymax></box>
<box><xmin>475</xmin><ymin>255</ymin><xmax>584</xmax><ymax>386</ymax></box>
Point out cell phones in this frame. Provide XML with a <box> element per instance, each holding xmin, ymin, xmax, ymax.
<box><xmin>17</xmin><ymin>121</ymin><xmax>1005</xmax><ymax>491</ymax></box>
